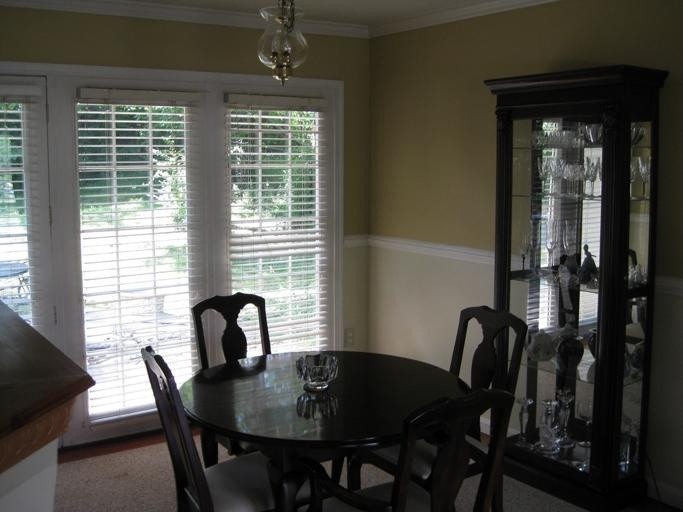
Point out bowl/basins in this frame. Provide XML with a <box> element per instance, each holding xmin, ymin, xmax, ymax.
<box><xmin>296</xmin><ymin>354</ymin><xmax>339</xmax><ymax>389</ymax></box>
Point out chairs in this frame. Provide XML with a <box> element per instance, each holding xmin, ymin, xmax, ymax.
<box><xmin>296</xmin><ymin>389</ymin><xmax>516</xmax><ymax>512</ymax></box>
<box><xmin>142</xmin><ymin>345</ymin><xmax>337</xmax><ymax>511</ymax></box>
<box><xmin>191</xmin><ymin>292</ymin><xmax>272</xmax><ymax>464</ymax></box>
<box><xmin>346</xmin><ymin>306</ymin><xmax>529</xmax><ymax>512</ymax></box>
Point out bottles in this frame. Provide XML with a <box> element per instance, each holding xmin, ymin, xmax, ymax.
<box><xmin>539</xmin><ymin>399</ymin><xmax>562</xmax><ymax>454</ymax></box>
<box><xmin>558</xmin><ymin>336</ymin><xmax>584</xmax><ymax>369</ymax></box>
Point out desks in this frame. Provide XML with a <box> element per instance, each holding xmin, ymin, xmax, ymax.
<box><xmin>180</xmin><ymin>351</ymin><xmax>473</xmax><ymax>512</ymax></box>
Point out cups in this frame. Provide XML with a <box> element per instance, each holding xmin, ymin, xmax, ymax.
<box><xmin>534</xmin><ymin>120</ymin><xmax>586</xmax><ymax>149</ymax></box>
<box><xmin>562</xmin><ymin>220</ymin><xmax>581</xmax><ymax>256</ymax></box>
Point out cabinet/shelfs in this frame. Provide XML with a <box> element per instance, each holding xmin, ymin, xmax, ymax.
<box><xmin>483</xmin><ymin>64</ymin><xmax>670</xmax><ymax>512</ymax></box>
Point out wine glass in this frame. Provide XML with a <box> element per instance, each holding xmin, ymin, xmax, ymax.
<box><xmin>516</xmin><ymin>218</ymin><xmax>559</xmax><ymax>274</ymax></box>
<box><xmin>629</xmin><ymin>156</ymin><xmax>650</xmax><ymax>200</ymax></box>
<box><xmin>534</xmin><ymin>153</ymin><xmax>598</xmax><ymax>197</ymax></box>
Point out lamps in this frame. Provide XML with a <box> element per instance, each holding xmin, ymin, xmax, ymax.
<box><xmin>256</xmin><ymin>0</ymin><xmax>310</xmax><ymax>87</ymax></box>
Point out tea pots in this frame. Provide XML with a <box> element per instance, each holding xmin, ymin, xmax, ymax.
<box><xmin>524</xmin><ymin>329</ymin><xmax>561</xmax><ymax>362</ymax></box>
